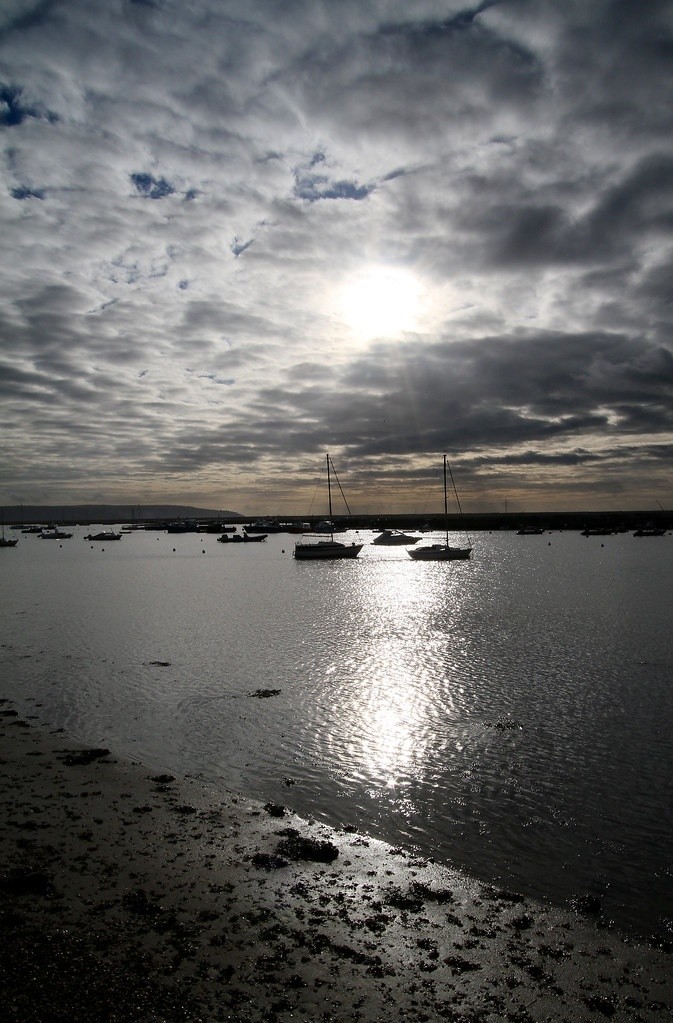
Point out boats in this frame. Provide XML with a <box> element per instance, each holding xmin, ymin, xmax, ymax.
<box><xmin>370</xmin><ymin>531</ymin><xmax>422</xmax><ymax>545</ymax></box>
<box><xmin>121</xmin><ymin>506</ymin><xmax>237</xmax><ymax>533</ymax></box>
<box><xmin>216</xmin><ymin>529</ymin><xmax>268</xmax><ymax>543</ymax></box>
<box><xmin>83</xmin><ymin>528</ymin><xmax>122</xmax><ymax>541</ymax></box>
<box><xmin>9</xmin><ymin>522</ymin><xmax>59</xmax><ymax>534</ymax></box>
<box><xmin>632</xmin><ymin>523</ymin><xmax>668</xmax><ymax>536</ymax></box>
<box><xmin>242</xmin><ymin>521</ymin><xmax>350</xmax><ymax>535</ymax></box>
<box><xmin>516</xmin><ymin>527</ymin><xmax>544</xmax><ymax>536</ymax></box>
<box><xmin>38</xmin><ymin>532</ymin><xmax>73</xmax><ymax>539</ymax></box>
<box><xmin>580</xmin><ymin>523</ymin><xmax>629</xmax><ymax>536</ymax></box>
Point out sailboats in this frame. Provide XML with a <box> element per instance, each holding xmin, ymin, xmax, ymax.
<box><xmin>0</xmin><ymin>514</ymin><xmax>19</xmax><ymax>547</ymax></box>
<box><xmin>294</xmin><ymin>453</ymin><xmax>365</xmax><ymax>560</ymax></box>
<box><xmin>403</xmin><ymin>455</ymin><xmax>476</xmax><ymax>560</ymax></box>
